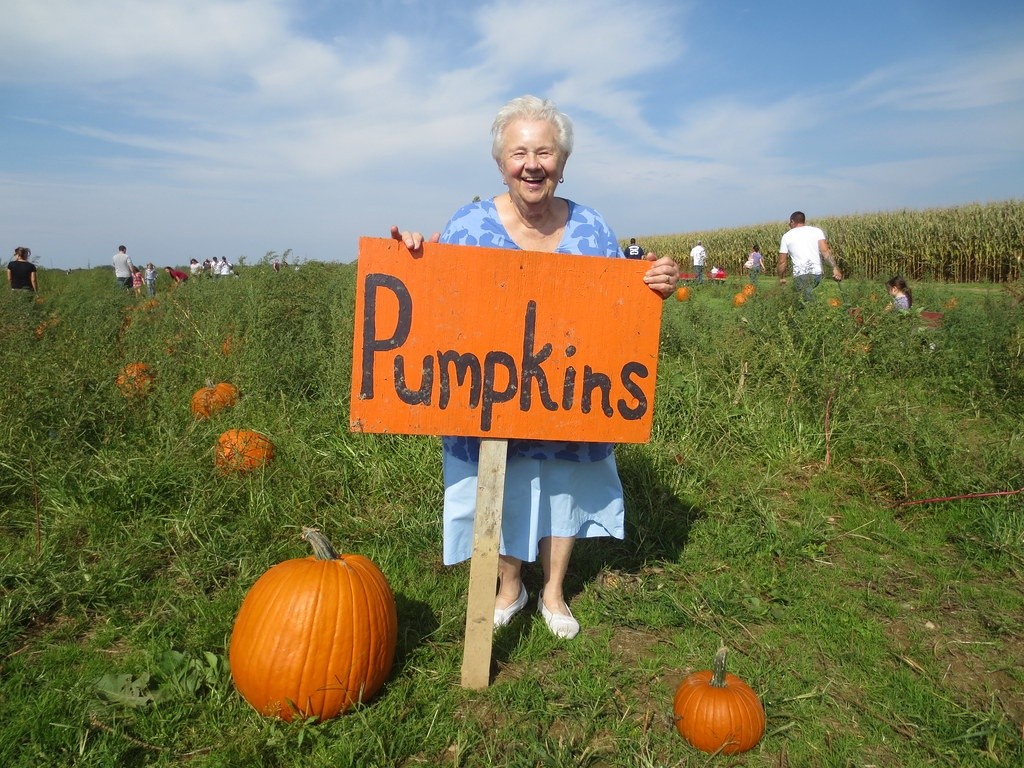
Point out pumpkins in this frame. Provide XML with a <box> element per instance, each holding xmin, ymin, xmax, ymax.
<box><xmin>828</xmin><ymin>299</ymin><xmax>839</xmax><ymax>307</ymax></box>
<box><xmin>677</xmin><ymin>284</ymin><xmax>691</xmax><ymax>302</ymax></box>
<box><xmin>673</xmin><ymin>644</ymin><xmax>766</xmax><ymax>755</ymax></box>
<box><xmin>116</xmin><ymin>363</ymin><xmax>272</xmax><ymax>476</ymax></box>
<box><xmin>733</xmin><ymin>283</ymin><xmax>755</xmax><ymax>307</ymax></box>
<box><xmin>229</xmin><ymin>526</ymin><xmax>397</xmax><ymax>723</ymax></box>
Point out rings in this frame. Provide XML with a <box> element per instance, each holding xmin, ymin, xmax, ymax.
<box><xmin>666</xmin><ymin>276</ymin><xmax>670</xmax><ymax>284</ymax></box>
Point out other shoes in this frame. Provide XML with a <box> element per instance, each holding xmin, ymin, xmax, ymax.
<box><xmin>493</xmin><ymin>582</ymin><xmax>529</xmax><ymax>629</ymax></box>
<box><xmin>537</xmin><ymin>588</ymin><xmax>580</xmax><ymax>640</ymax></box>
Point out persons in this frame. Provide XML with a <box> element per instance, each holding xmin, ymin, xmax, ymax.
<box><xmin>778</xmin><ymin>211</ymin><xmax>843</xmax><ymax>302</ymax></box>
<box><xmin>885</xmin><ymin>276</ymin><xmax>914</xmax><ymax>314</ymax></box>
<box><xmin>7</xmin><ymin>246</ymin><xmax>40</xmax><ymax>300</ymax></box>
<box><xmin>624</xmin><ymin>238</ymin><xmax>767</xmax><ymax>285</ymax></box>
<box><xmin>112</xmin><ymin>245</ymin><xmax>300</xmax><ymax>298</ymax></box>
<box><xmin>389</xmin><ymin>94</ymin><xmax>681</xmax><ymax>640</ymax></box>
